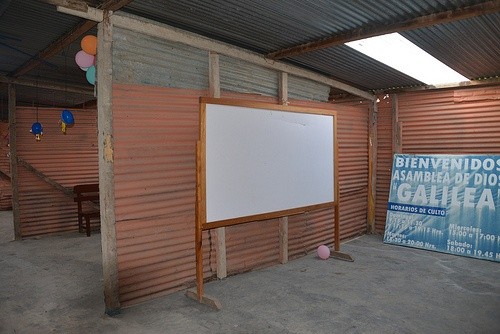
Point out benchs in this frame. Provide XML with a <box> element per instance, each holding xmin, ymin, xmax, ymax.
<box><xmin>73</xmin><ymin>183</ymin><xmax>101</xmax><ymax>236</ymax></box>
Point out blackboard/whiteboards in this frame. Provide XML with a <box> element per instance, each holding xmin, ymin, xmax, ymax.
<box><xmin>199</xmin><ymin>95</ymin><xmax>339</xmax><ymax>230</ymax></box>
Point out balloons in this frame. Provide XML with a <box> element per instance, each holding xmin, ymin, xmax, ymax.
<box><xmin>317</xmin><ymin>244</ymin><xmax>331</xmax><ymax>260</ymax></box>
<box><xmin>75</xmin><ymin>49</ymin><xmax>96</xmax><ymax>67</ymax></box>
<box><xmin>81</xmin><ymin>35</ymin><xmax>98</xmax><ymax>56</ymax></box>
<box><xmin>59</xmin><ymin>109</ymin><xmax>75</xmax><ymax>133</ymax></box>
<box><xmin>30</xmin><ymin>122</ymin><xmax>43</xmax><ymax>142</ymax></box>
<box><xmin>85</xmin><ymin>65</ymin><xmax>97</xmax><ymax>85</ymax></box>
